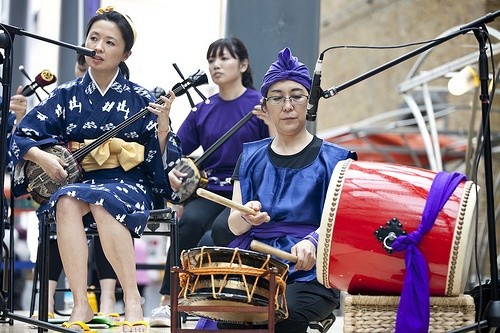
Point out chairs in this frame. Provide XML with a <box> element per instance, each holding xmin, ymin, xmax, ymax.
<box><xmin>29</xmin><ymin>199</ymin><xmax>179</xmax><ymax>333</ymax></box>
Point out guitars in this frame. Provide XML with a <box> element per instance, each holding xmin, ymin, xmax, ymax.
<box><xmin>27</xmin><ymin>62</ymin><xmax>211</xmax><ymax>206</ymax></box>
<box><xmin>167</xmin><ymin>102</ymin><xmax>265</xmax><ymax>204</ymax></box>
<box><xmin>0</xmin><ymin>66</ymin><xmax>57</xmax><ymax>124</ymax></box>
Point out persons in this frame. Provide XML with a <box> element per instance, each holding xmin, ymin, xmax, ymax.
<box><xmin>10</xmin><ymin>10</ymin><xmax>182</xmax><ymax>332</ymax></box>
<box><xmin>27</xmin><ymin>42</ymin><xmax>139</xmax><ymax>329</ymax></box>
<box><xmin>149</xmin><ymin>38</ymin><xmax>278</xmax><ymax>325</ymax></box>
<box><xmin>194</xmin><ymin>48</ymin><xmax>357</xmax><ymax>331</ymax></box>
<box><xmin>1</xmin><ymin>81</ymin><xmax>27</xmax><ymax>221</ymax></box>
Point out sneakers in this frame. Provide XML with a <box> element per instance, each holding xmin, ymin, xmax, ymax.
<box><xmin>149</xmin><ymin>305</ymin><xmax>171</xmax><ymax>327</ymax></box>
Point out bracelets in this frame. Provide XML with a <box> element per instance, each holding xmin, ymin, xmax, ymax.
<box><xmin>157</xmin><ymin>127</ymin><xmax>170</xmax><ymax>133</ymax></box>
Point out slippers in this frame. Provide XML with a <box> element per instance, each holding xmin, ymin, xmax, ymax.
<box><xmin>97</xmin><ymin>312</ymin><xmax>121</xmax><ymax>326</ymax></box>
<box><xmin>122</xmin><ymin>319</ymin><xmax>151</xmax><ymax>333</ymax></box>
<box><xmin>53</xmin><ymin>321</ymin><xmax>99</xmax><ymax>333</ymax></box>
<box><xmin>30</xmin><ymin>311</ymin><xmax>55</xmax><ymax>329</ymax></box>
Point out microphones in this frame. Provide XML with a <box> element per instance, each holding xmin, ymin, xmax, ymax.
<box><xmin>305</xmin><ymin>52</ymin><xmax>324</xmax><ymax>121</ymax></box>
<box><xmin>20</xmin><ymin>69</ymin><xmax>58</xmax><ymax>96</ymax></box>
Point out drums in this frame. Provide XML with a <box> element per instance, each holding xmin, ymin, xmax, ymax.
<box><xmin>315</xmin><ymin>158</ymin><xmax>481</xmax><ymax>299</ymax></box>
<box><xmin>173</xmin><ymin>246</ymin><xmax>289</xmax><ymax>324</ymax></box>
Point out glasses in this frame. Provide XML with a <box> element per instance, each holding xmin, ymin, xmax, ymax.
<box><xmin>266</xmin><ymin>93</ymin><xmax>309</xmax><ymax>105</ymax></box>
